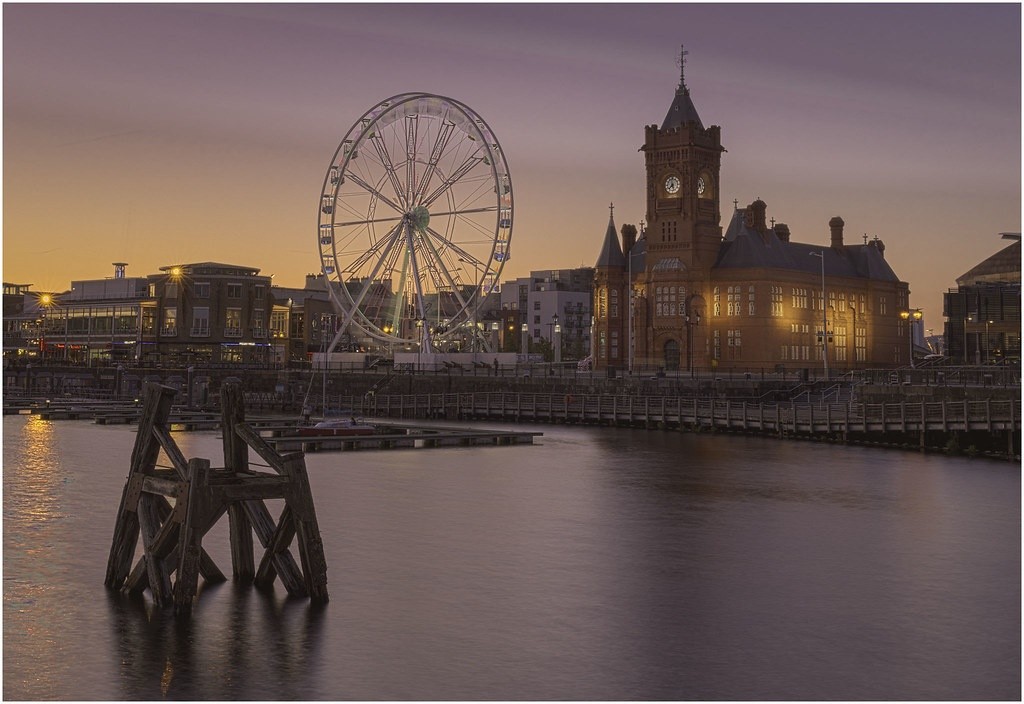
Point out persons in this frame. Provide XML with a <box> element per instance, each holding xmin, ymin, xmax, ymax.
<box><xmin>349</xmin><ymin>417</ymin><xmax>357</xmax><ymax>426</ymax></box>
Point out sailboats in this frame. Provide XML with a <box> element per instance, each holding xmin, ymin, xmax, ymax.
<box><xmin>283</xmin><ymin>312</ymin><xmax>378</xmax><ymax>436</ymax></box>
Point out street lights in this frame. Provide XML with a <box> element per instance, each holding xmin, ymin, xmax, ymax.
<box><xmin>809</xmin><ymin>251</ymin><xmax>828</xmax><ymax>379</ymax></box>
<box><xmin>685</xmin><ymin>312</ymin><xmax>700</xmax><ymax>379</ymax></box>
<box><xmin>963</xmin><ymin>315</ymin><xmax>972</xmax><ymax>365</ymax></box>
<box><xmin>899</xmin><ymin>307</ymin><xmax>923</xmax><ymax>369</ymax></box>
<box><xmin>985</xmin><ymin>320</ymin><xmax>993</xmax><ymax>365</ymax></box>
<box><xmin>552</xmin><ymin>312</ymin><xmax>558</xmax><ymax>362</ymax></box>
